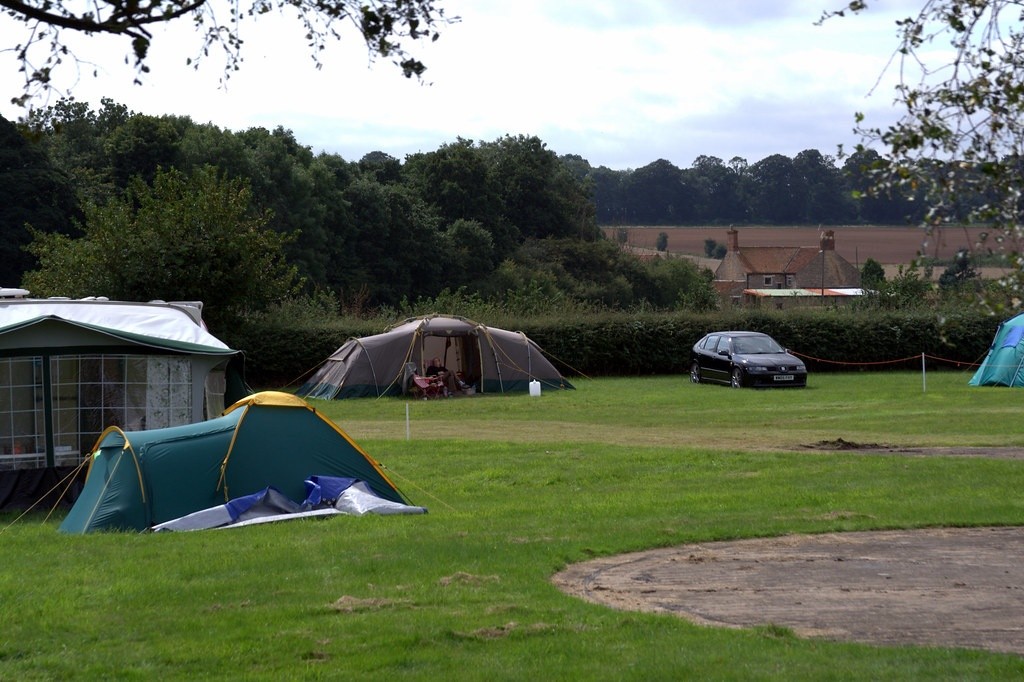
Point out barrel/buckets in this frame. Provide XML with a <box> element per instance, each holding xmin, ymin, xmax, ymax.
<box><xmin>529</xmin><ymin>379</ymin><xmax>541</xmax><ymax>396</ymax></box>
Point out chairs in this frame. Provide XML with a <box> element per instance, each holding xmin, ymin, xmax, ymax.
<box><xmin>413</xmin><ymin>372</ymin><xmax>461</xmax><ymax>399</ymax></box>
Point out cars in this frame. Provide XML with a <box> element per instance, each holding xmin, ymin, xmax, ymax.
<box><xmin>690</xmin><ymin>331</ymin><xmax>807</xmax><ymax>388</ymax></box>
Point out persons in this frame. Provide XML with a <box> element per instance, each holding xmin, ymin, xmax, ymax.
<box><xmin>426</xmin><ymin>357</ymin><xmax>470</xmax><ymax>397</ymax></box>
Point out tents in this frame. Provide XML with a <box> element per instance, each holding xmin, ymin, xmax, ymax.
<box><xmin>0</xmin><ymin>288</ymin><xmax>255</xmax><ymax>515</ymax></box>
<box><xmin>55</xmin><ymin>391</ymin><xmax>429</xmax><ymax>535</ymax></box>
<box><xmin>967</xmin><ymin>310</ymin><xmax>1024</xmax><ymax>388</ymax></box>
<box><xmin>293</xmin><ymin>312</ymin><xmax>577</xmax><ymax>400</ymax></box>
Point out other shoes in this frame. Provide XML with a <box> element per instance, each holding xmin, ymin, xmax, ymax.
<box><xmin>461</xmin><ymin>384</ymin><xmax>471</xmax><ymax>391</ymax></box>
<box><xmin>448</xmin><ymin>392</ymin><xmax>453</xmax><ymax>397</ymax></box>
